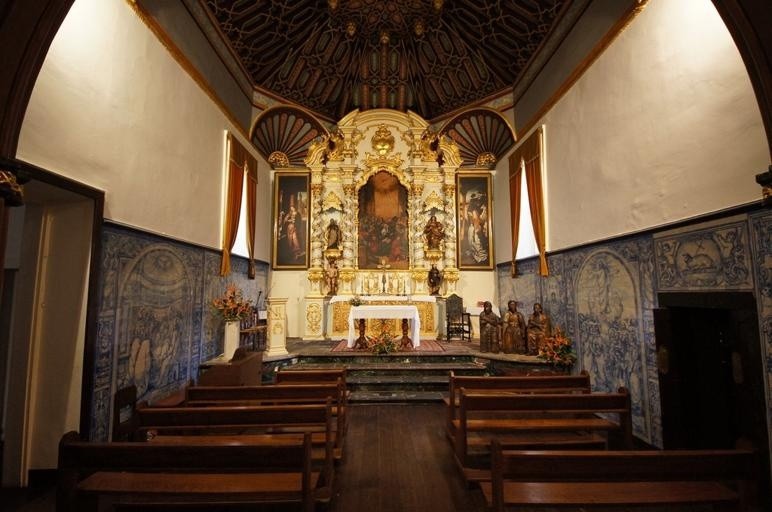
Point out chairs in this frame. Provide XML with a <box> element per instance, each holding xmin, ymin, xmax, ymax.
<box><xmin>445</xmin><ymin>293</ymin><xmax>472</xmax><ymax>343</ymax></box>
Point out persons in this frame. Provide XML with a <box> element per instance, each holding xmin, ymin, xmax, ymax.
<box><xmin>527</xmin><ymin>302</ymin><xmax>551</xmax><ymax>358</ymax></box>
<box><xmin>422</xmin><ymin>216</ymin><xmax>448</xmax><ymax>251</ymax></box>
<box><xmin>500</xmin><ymin>300</ymin><xmax>528</xmax><ymax>356</ymax></box>
<box><xmin>426</xmin><ymin>261</ymin><xmax>444</xmax><ymax>296</ymax></box>
<box><xmin>327</xmin><ymin>218</ymin><xmax>342</xmax><ymax>251</ymax></box>
<box><xmin>326</xmin><ymin>263</ymin><xmax>340</xmax><ymax>294</ymax></box>
<box><xmin>479</xmin><ymin>301</ymin><xmax>503</xmax><ymax>353</ymax></box>
<box><xmin>359</xmin><ymin>214</ymin><xmax>408</xmax><ymax>267</ymax></box>
<box><xmin>465</xmin><ymin>207</ymin><xmax>488</xmax><ymax>262</ymax></box>
<box><xmin>282</xmin><ymin>205</ymin><xmax>303</xmax><ymax>263</ymax></box>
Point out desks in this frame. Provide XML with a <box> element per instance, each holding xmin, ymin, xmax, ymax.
<box><xmin>349</xmin><ymin>305</ymin><xmax>419</xmax><ymax>352</ymax></box>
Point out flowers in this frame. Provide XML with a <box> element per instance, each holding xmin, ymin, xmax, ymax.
<box><xmin>347</xmin><ymin>293</ymin><xmax>365</xmax><ymax>307</ymax></box>
<box><xmin>208</xmin><ymin>281</ymin><xmax>256</xmax><ymax>321</ymax></box>
<box><xmin>364</xmin><ymin>321</ymin><xmax>401</xmax><ymax>352</ymax></box>
<box><xmin>536</xmin><ymin>325</ymin><xmax>576</xmax><ymax>369</ymax></box>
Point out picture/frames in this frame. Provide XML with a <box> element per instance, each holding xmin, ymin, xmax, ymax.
<box><xmin>455</xmin><ymin>172</ymin><xmax>496</xmax><ymax>272</ymax></box>
<box><xmin>270</xmin><ymin>171</ymin><xmax>312</xmax><ymax>270</ymax></box>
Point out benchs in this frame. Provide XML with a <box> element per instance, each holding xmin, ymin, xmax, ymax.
<box><xmin>54</xmin><ymin>362</ymin><xmax>350</xmax><ymax>510</ymax></box>
<box><xmin>476</xmin><ymin>440</ymin><xmax>770</xmax><ymax>510</ymax></box>
<box><xmin>439</xmin><ymin>368</ymin><xmax>633</xmax><ymax>489</ymax></box>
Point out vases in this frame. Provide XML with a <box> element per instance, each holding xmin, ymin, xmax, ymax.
<box><xmin>224</xmin><ymin>321</ymin><xmax>240</xmax><ymax>361</ymax></box>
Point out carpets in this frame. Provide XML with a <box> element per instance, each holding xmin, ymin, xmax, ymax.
<box><xmin>330</xmin><ymin>338</ymin><xmax>447</xmax><ymax>352</ymax></box>
